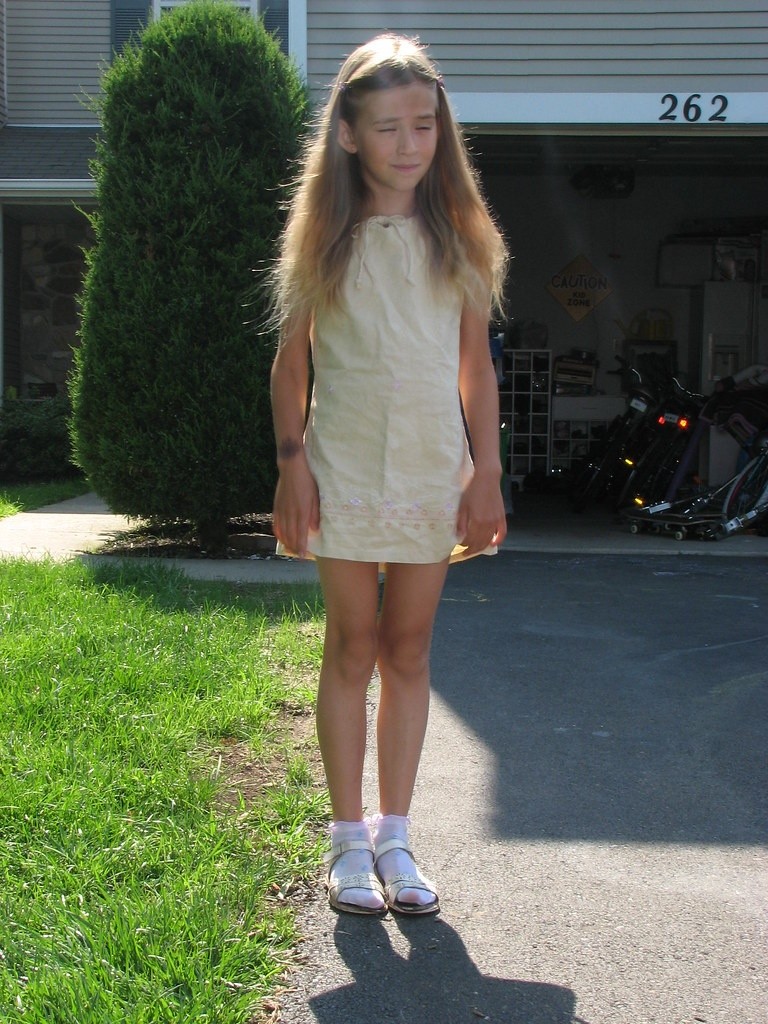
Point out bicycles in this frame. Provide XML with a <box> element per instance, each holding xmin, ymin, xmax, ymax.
<box><xmin>564</xmin><ymin>354</ymin><xmax>768</xmax><ymax>542</ymax></box>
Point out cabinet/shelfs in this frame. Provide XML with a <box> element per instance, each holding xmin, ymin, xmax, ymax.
<box><xmin>491</xmin><ymin>348</ymin><xmax>553</xmax><ymax>493</ymax></box>
<box><xmin>550</xmin><ymin>393</ymin><xmax>631</xmax><ymax>479</ymax></box>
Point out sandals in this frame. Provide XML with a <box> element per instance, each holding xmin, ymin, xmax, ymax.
<box><xmin>323</xmin><ymin>841</ymin><xmax>388</xmax><ymax>914</ymax></box>
<box><xmin>373</xmin><ymin>839</ymin><xmax>440</xmax><ymax>915</ymax></box>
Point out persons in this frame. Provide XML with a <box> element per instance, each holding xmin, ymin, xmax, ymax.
<box><xmin>271</xmin><ymin>33</ymin><xmax>508</xmax><ymax>917</ymax></box>
